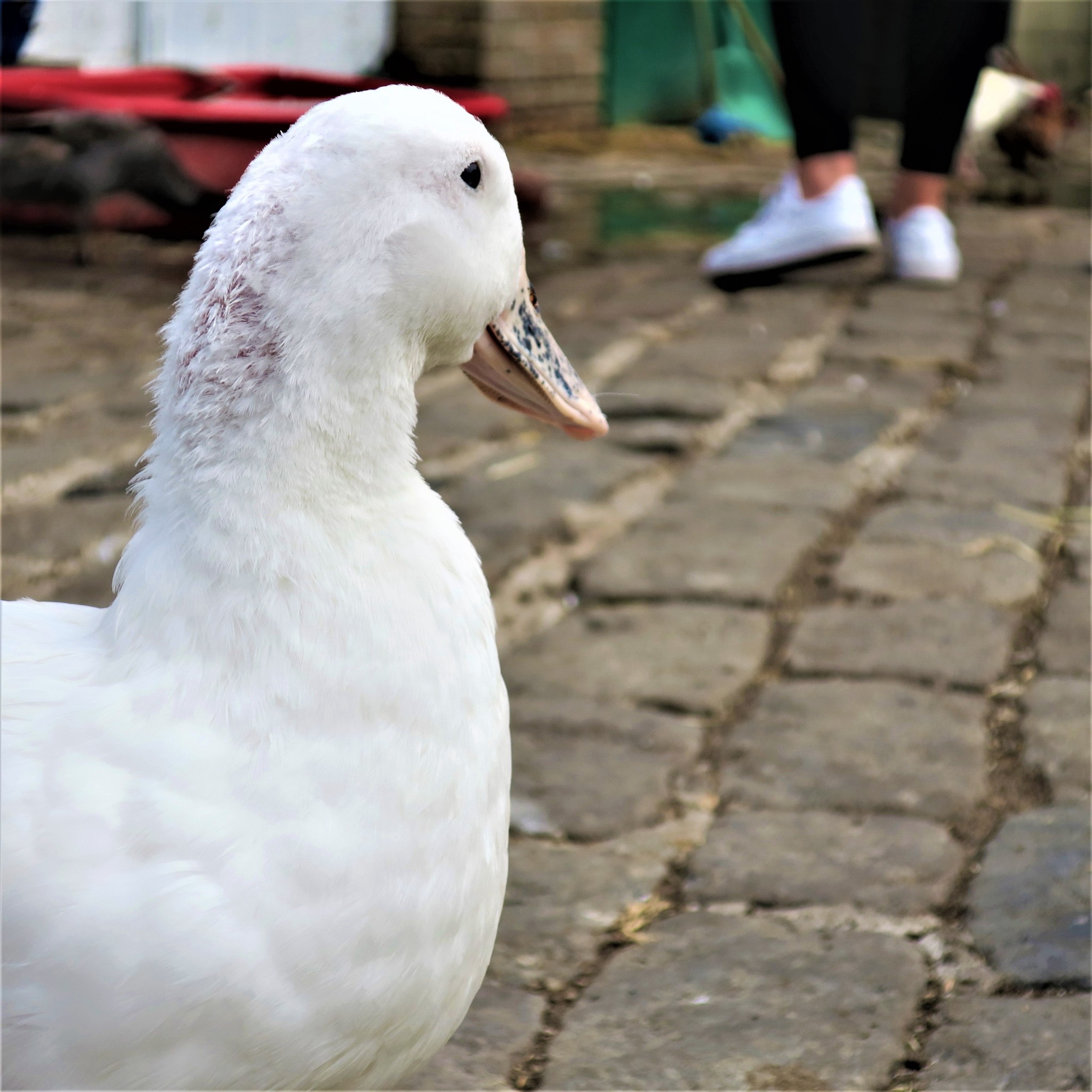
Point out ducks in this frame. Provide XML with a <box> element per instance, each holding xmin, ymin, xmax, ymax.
<box><xmin>1</xmin><ymin>84</ymin><xmax>612</xmax><ymax>1092</ymax></box>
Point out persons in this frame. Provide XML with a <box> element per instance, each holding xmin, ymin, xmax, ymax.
<box><xmin>699</xmin><ymin>0</ymin><xmax>1009</xmax><ymax>284</ymax></box>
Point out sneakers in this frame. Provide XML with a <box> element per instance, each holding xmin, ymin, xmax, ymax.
<box><xmin>694</xmin><ymin>171</ymin><xmax>885</xmax><ymax>293</ymax></box>
<box><xmin>881</xmin><ymin>200</ymin><xmax>965</xmax><ymax>291</ymax></box>
